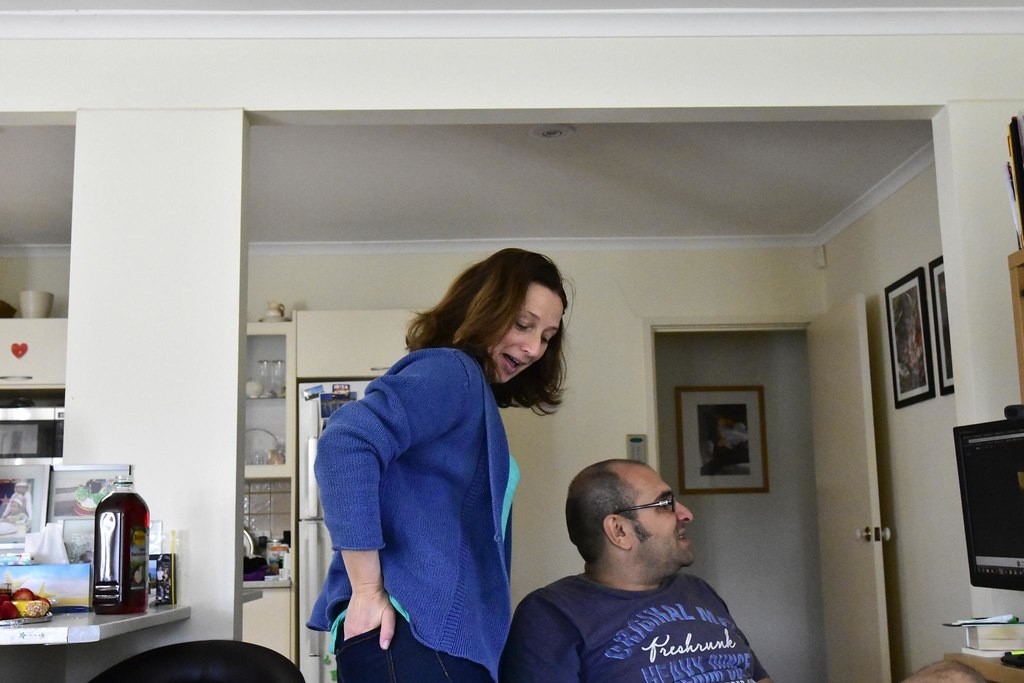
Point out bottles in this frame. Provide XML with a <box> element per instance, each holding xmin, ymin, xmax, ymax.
<box><xmin>257</xmin><ymin>360</ymin><xmax>286</xmax><ymax>398</ymax></box>
<box><xmin>245</xmin><ymin>378</ymin><xmax>263</xmax><ymax>398</ymax></box>
<box><xmin>12</xmin><ymin>397</ymin><xmax>35</xmax><ymax>408</ymax></box>
<box><xmin>92</xmin><ymin>474</ymin><xmax>150</xmax><ymax>614</ymax></box>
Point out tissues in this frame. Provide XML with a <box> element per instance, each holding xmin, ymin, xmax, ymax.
<box><xmin>0</xmin><ymin>517</ymin><xmax>91</xmax><ymax>611</ymax></box>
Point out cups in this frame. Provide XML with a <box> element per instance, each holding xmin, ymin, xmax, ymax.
<box><xmin>19</xmin><ymin>289</ymin><xmax>54</xmax><ymax>318</ymax></box>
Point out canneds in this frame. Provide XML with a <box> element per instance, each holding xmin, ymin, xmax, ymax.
<box><xmin>269</xmin><ymin>543</ymin><xmax>289</xmax><ymax>574</ymax></box>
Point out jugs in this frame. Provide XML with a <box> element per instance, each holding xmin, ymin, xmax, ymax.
<box><xmin>266</xmin><ymin>300</ymin><xmax>285</xmax><ymax>318</ymax></box>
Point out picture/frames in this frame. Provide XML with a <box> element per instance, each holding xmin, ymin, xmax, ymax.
<box><xmin>673</xmin><ymin>385</ymin><xmax>769</xmax><ymax>494</ymax></box>
<box><xmin>928</xmin><ymin>256</ymin><xmax>954</xmax><ymax>396</ymax></box>
<box><xmin>885</xmin><ymin>267</ymin><xmax>936</xmax><ymax>410</ymax></box>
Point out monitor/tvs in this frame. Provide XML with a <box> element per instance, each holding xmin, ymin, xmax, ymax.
<box><xmin>953</xmin><ymin>416</ymin><xmax>1024</xmax><ymax>592</ymax></box>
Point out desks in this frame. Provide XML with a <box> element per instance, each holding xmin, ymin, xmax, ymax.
<box><xmin>942</xmin><ymin>652</ymin><xmax>1024</xmax><ymax>683</ymax></box>
<box><xmin>0</xmin><ymin>605</ymin><xmax>191</xmax><ymax>683</ymax></box>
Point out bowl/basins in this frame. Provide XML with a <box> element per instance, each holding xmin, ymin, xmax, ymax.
<box><xmin>0</xmin><ymin>299</ymin><xmax>17</xmax><ymax>318</ymax></box>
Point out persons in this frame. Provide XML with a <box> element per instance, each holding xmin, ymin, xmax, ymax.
<box><xmin>498</xmin><ymin>460</ymin><xmax>985</xmax><ymax>683</ymax></box>
<box><xmin>305</xmin><ymin>248</ymin><xmax>568</xmax><ymax>683</ymax></box>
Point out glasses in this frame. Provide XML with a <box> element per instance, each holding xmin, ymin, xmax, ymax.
<box><xmin>613</xmin><ymin>495</ymin><xmax>676</xmax><ymax>514</ymax></box>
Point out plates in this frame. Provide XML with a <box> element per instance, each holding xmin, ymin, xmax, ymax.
<box><xmin>260</xmin><ymin>317</ymin><xmax>290</xmax><ymax>322</ymax></box>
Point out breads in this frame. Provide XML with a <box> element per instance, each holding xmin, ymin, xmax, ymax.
<box><xmin>267</xmin><ymin>449</ymin><xmax>285</xmax><ymax>464</ymax></box>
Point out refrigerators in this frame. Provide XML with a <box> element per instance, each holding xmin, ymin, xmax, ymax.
<box><xmin>296</xmin><ymin>380</ymin><xmax>370</xmax><ymax>683</ymax></box>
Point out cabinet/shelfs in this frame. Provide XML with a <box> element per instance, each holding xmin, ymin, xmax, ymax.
<box><xmin>239</xmin><ymin>322</ymin><xmax>297</xmax><ymax>663</ymax></box>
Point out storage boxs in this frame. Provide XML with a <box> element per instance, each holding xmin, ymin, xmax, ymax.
<box><xmin>0</xmin><ymin>563</ymin><xmax>94</xmax><ymax>612</ymax></box>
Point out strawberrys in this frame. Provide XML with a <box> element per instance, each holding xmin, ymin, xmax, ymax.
<box><xmin>0</xmin><ymin>588</ymin><xmax>50</xmax><ymax>621</ymax></box>
